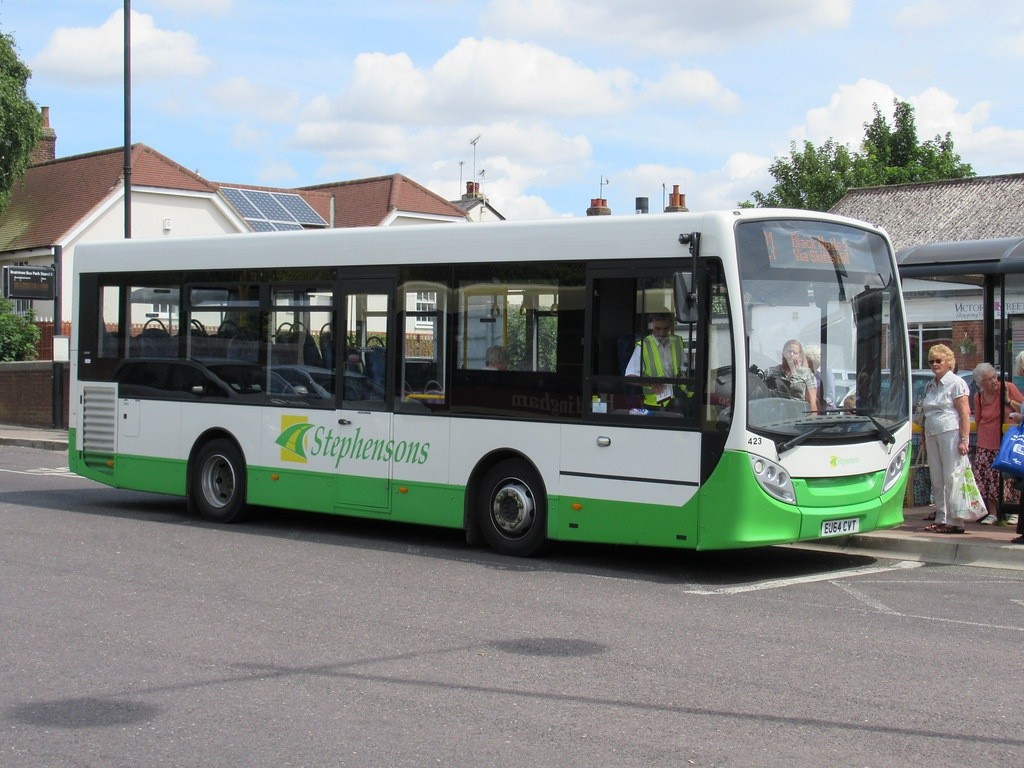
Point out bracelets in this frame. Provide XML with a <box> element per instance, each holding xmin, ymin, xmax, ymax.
<box><xmin>1008</xmin><ymin>400</ymin><xmax>1011</xmax><ymax>405</ymax></box>
<box><xmin>960</xmin><ymin>437</ymin><xmax>969</xmax><ymax>443</ymax></box>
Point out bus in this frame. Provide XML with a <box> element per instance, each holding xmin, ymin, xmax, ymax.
<box><xmin>68</xmin><ymin>207</ymin><xmax>912</xmax><ymax>557</ymax></box>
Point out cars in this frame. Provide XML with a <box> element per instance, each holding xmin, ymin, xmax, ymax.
<box><xmin>826</xmin><ymin>370</ymin><xmax>1022</xmax><ymax>422</ymax></box>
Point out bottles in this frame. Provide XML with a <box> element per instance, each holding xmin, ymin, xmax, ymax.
<box><xmin>629</xmin><ymin>408</ymin><xmax>653</xmax><ymax>417</ymax></box>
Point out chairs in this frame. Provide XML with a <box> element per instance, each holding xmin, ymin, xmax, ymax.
<box><xmin>102</xmin><ymin>317</ymin><xmax>445</xmax><ymax>411</ymax></box>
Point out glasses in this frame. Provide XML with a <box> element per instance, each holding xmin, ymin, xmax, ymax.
<box><xmin>654</xmin><ymin>325</ymin><xmax>672</xmax><ymax>331</ymax></box>
<box><xmin>929</xmin><ymin>358</ymin><xmax>941</xmax><ymax>365</ymax></box>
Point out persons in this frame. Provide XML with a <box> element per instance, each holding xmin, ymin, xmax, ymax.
<box><xmin>1011</xmin><ymin>351</ymin><xmax>1024</xmax><ymax>544</ymax></box>
<box><xmin>803</xmin><ymin>346</ymin><xmax>836</xmax><ymax>410</ymax></box>
<box><xmin>763</xmin><ymin>339</ymin><xmax>818</xmax><ymax>416</ymax></box>
<box><xmin>972</xmin><ymin>363</ymin><xmax>1024</xmax><ymax>525</ymax></box>
<box><xmin>477</xmin><ymin>346</ymin><xmax>508</xmax><ymax>370</ymax></box>
<box><xmin>920</xmin><ymin>344</ymin><xmax>971</xmax><ymax>533</ymax></box>
<box><xmin>624</xmin><ymin>307</ymin><xmax>694</xmax><ymax>407</ymax></box>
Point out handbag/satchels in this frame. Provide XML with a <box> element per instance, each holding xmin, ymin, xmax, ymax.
<box><xmin>949</xmin><ymin>453</ymin><xmax>988</xmax><ymax>521</ymax></box>
<box><xmin>991</xmin><ymin>415</ymin><xmax>1024</xmax><ymax>475</ymax></box>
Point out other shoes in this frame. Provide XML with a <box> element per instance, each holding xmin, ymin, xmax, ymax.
<box><xmin>923</xmin><ymin>511</ymin><xmax>936</xmax><ymax>520</ymax></box>
<box><xmin>926</xmin><ymin>524</ymin><xmax>964</xmax><ymax>533</ymax></box>
<box><xmin>981</xmin><ymin>515</ymin><xmax>997</xmax><ymax>524</ymax></box>
<box><xmin>1006</xmin><ymin>514</ymin><xmax>1018</xmax><ymax>524</ymax></box>
<box><xmin>1011</xmin><ymin>535</ymin><xmax>1024</xmax><ymax>544</ymax></box>
